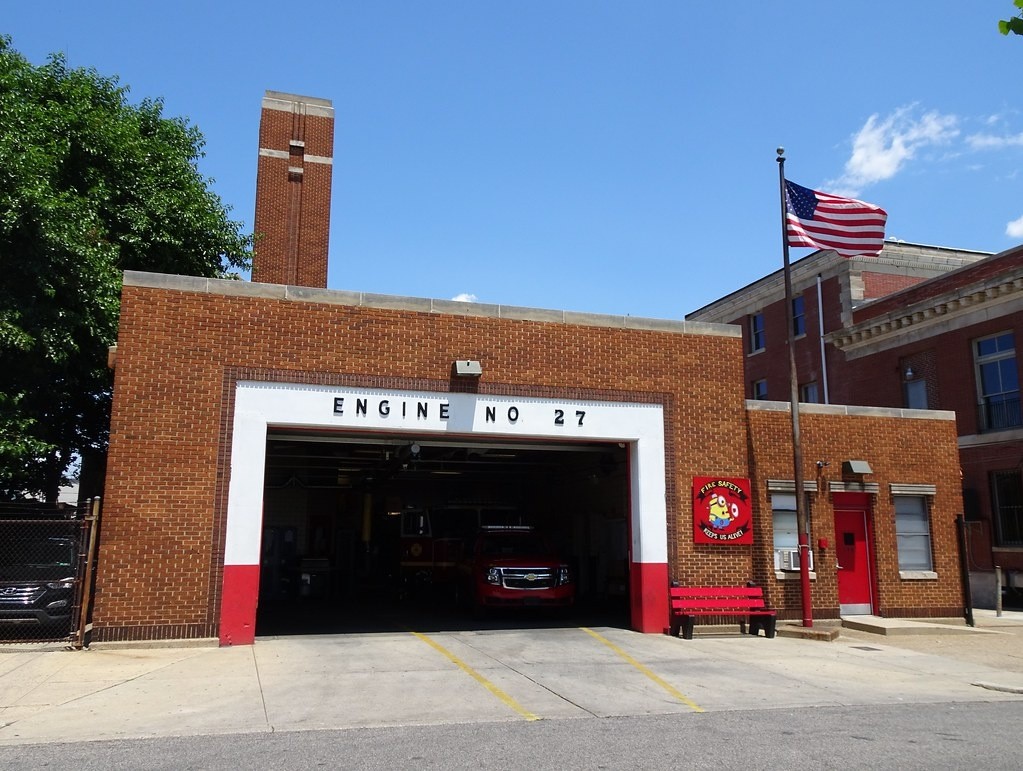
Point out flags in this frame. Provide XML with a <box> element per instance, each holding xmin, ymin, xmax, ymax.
<box><xmin>785</xmin><ymin>178</ymin><xmax>887</xmax><ymax>257</ymax></box>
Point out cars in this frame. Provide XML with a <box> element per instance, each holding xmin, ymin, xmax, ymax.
<box><xmin>0</xmin><ymin>535</ymin><xmax>100</xmax><ymax>637</ymax></box>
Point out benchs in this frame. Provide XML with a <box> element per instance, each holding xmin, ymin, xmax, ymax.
<box><xmin>670</xmin><ymin>580</ymin><xmax>778</xmax><ymax>640</ymax></box>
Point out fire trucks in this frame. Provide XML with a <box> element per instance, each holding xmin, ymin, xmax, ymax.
<box><xmin>396</xmin><ymin>498</ymin><xmax>580</xmax><ymax>621</ymax></box>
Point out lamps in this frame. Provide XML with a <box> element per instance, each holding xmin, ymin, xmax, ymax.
<box><xmin>905</xmin><ymin>367</ymin><xmax>914</xmax><ymax>381</ymax></box>
<box><xmin>288</xmin><ymin>166</ymin><xmax>304</xmax><ymax>176</ymax></box>
<box><xmin>290</xmin><ymin>140</ymin><xmax>306</xmax><ymax>148</ymax></box>
<box><xmin>456</xmin><ymin>360</ymin><xmax>483</xmax><ymax>376</ymax></box>
